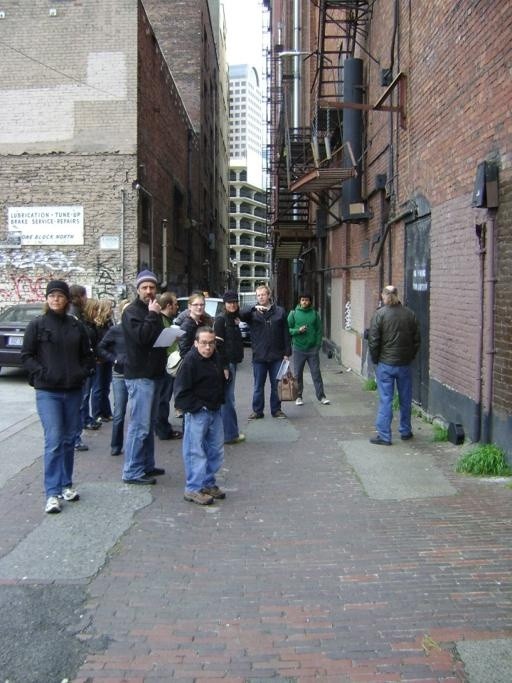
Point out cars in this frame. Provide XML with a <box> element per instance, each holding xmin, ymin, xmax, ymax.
<box><xmin>172</xmin><ymin>297</ymin><xmax>226</xmax><ymax>320</ymax></box>
<box><xmin>238</xmin><ymin>322</ymin><xmax>252</xmax><ymax>346</ymax></box>
<box><xmin>0</xmin><ymin>302</ymin><xmax>49</xmax><ymax>372</ymax></box>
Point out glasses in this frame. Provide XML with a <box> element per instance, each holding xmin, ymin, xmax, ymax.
<box><xmin>202</xmin><ymin>341</ymin><xmax>216</xmax><ymax>346</ymax></box>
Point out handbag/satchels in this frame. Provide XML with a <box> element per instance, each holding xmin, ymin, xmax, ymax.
<box><xmin>277</xmin><ymin>366</ymin><xmax>298</xmax><ymax>401</ymax></box>
<box><xmin>165</xmin><ymin>351</ymin><xmax>184</xmax><ymax>377</ymax></box>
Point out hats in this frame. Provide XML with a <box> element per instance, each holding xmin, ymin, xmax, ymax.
<box><xmin>137</xmin><ymin>270</ymin><xmax>158</xmax><ymax>289</ymax></box>
<box><xmin>46</xmin><ymin>281</ymin><xmax>69</xmax><ymax>298</ymax></box>
<box><xmin>223</xmin><ymin>291</ymin><xmax>239</xmax><ymax>302</ymax></box>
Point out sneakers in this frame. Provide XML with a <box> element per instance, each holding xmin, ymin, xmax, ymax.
<box><xmin>275</xmin><ymin>409</ymin><xmax>287</xmax><ymax>418</ymax></box>
<box><xmin>74</xmin><ymin>442</ymin><xmax>88</xmax><ymax>451</ymax></box>
<box><xmin>247</xmin><ymin>411</ymin><xmax>261</xmax><ymax>420</ymax></box>
<box><xmin>111</xmin><ymin>445</ymin><xmax>121</xmax><ymax>455</ymax></box>
<box><xmin>57</xmin><ymin>487</ymin><xmax>79</xmax><ymax>500</ymax></box>
<box><xmin>184</xmin><ymin>485</ymin><xmax>225</xmax><ymax>504</ymax></box>
<box><xmin>320</xmin><ymin>398</ymin><xmax>330</xmax><ymax>405</ymax></box>
<box><xmin>295</xmin><ymin>397</ymin><xmax>304</xmax><ymax>405</ymax></box>
<box><xmin>45</xmin><ymin>496</ymin><xmax>61</xmax><ymax>514</ymax></box>
<box><xmin>226</xmin><ymin>434</ymin><xmax>245</xmax><ymax>443</ymax></box>
<box><xmin>124</xmin><ymin>468</ymin><xmax>164</xmax><ymax>484</ymax></box>
<box><xmin>169</xmin><ymin>431</ymin><xmax>182</xmax><ymax>439</ymax></box>
<box><xmin>86</xmin><ymin>414</ymin><xmax>113</xmax><ymax>429</ymax></box>
<box><xmin>401</xmin><ymin>432</ymin><xmax>413</xmax><ymax>439</ymax></box>
<box><xmin>370</xmin><ymin>436</ymin><xmax>391</xmax><ymax>444</ymax></box>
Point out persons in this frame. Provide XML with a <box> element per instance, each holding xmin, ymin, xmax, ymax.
<box><xmin>213</xmin><ymin>292</ymin><xmax>246</xmax><ymax>444</ymax></box>
<box><xmin>237</xmin><ymin>285</ymin><xmax>292</xmax><ymax>420</ymax></box>
<box><xmin>21</xmin><ymin>280</ymin><xmax>97</xmax><ymax>513</ymax></box>
<box><xmin>65</xmin><ymin>284</ymin><xmax>215</xmax><ymax>456</ymax></box>
<box><xmin>120</xmin><ymin>268</ymin><xmax>166</xmax><ymax>484</ymax></box>
<box><xmin>368</xmin><ymin>285</ymin><xmax>421</xmax><ymax>445</ymax></box>
<box><xmin>173</xmin><ymin>326</ymin><xmax>226</xmax><ymax>505</ymax></box>
<box><xmin>286</xmin><ymin>293</ymin><xmax>332</xmax><ymax>406</ymax></box>
